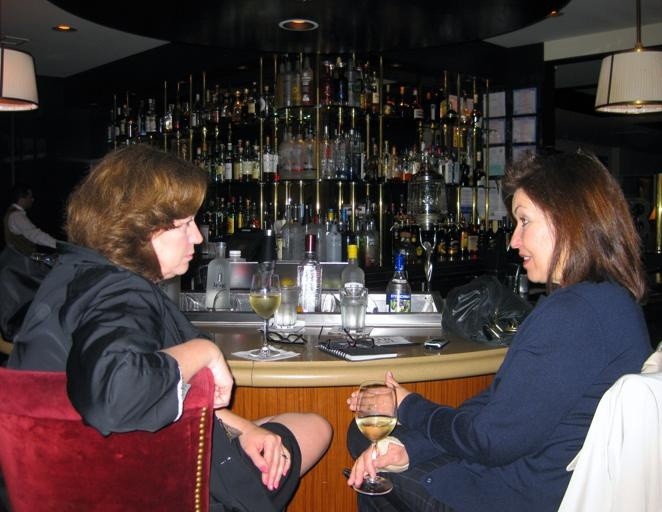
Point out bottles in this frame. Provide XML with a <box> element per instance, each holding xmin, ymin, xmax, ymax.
<box><xmin>103</xmin><ymin>52</ymin><xmax>528</xmax><ymax>331</ymax></box>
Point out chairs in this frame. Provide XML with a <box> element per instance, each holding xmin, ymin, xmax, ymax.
<box><xmin>558</xmin><ymin>345</ymin><xmax>662</xmax><ymax>512</ymax></box>
<box><xmin>0</xmin><ymin>367</ymin><xmax>215</xmax><ymax>512</ymax></box>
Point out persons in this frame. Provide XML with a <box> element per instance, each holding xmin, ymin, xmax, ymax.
<box><xmin>3</xmin><ymin>187</ymin><xmax>56</xmax><ymax>256</ymax></box>
<box><xmin>346</xmin><ymin>142</ymin><xmax>656</xmax><ymax>511</ymax></box>
<box><xmin>5</xmin><ymin>142</ymin><xmax>333</xmax><ymax>512</ymax></box>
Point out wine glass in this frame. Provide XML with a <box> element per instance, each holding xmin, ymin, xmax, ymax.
<box><xmin>345</xmin><ymin>380</ymin><xmax>398</xmax><ymax>497</ymax></box>
<box><xmin>249</xmin><ymin>266</ymin><xmax>283</xmax><ymax>359</ymax></box>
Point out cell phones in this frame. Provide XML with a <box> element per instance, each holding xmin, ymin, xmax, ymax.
<box><xmin>423</xmin><ymin>336</ymin><xmax>449</xmax><ymax>350</ymax></box>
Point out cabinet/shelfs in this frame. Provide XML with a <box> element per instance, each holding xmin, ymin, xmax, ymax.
<box><xmin>111</xmin><ymin>51</ymin><xmax>489</xmax><ymax>266</ymax></box>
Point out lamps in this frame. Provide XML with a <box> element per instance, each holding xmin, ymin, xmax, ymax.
<box><xmin>0</xmin><ymin>47</ymin><xmax>39</xmax><ymax>112</ymax></box>
<box><xmin>595</xmin><ymin>0</ymin><xmax>662</xmax><ymax>114</ymax></box>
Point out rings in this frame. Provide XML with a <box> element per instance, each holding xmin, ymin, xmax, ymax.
<box><xmin>368</xmin><ymin>403</ymin><xmax>372</xmax><ymax>410</ymax></box>
<box><xmin>280</xmin><ymin>454</ymin><xmax>287</xmax><ymax>459</ymax></box>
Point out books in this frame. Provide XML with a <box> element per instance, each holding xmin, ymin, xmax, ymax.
<box><xmin>318</xmin><ymin>339</ymin><xmax>397</xmax><ymax>361</ymax></box>
